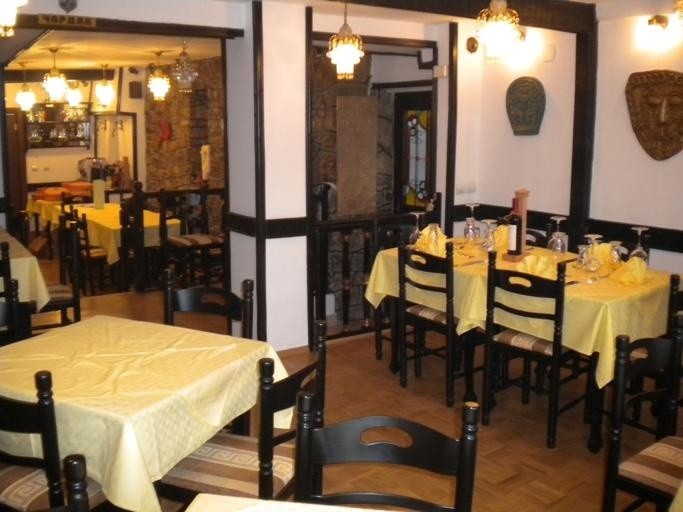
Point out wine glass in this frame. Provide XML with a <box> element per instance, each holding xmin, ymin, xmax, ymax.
<box><xmin>409</xmin><ymin>202</ymin><xmax>505</xmax><ymax>263</ymax></box>
<box><xmin>546</xmin><ymin>215</ymin><xmax>650</xmax><ymax>284</ymax></box>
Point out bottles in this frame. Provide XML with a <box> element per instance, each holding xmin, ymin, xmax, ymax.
<box><xmin>507</xmin><ymin>197</ymin><xmax>521</xmax><ymax>255</ymax></box>
<box><xmin>92</xmin><ymin>178</ymin><xmax>105</xmax><ymax>210</ymax></box>
<box><xmin>25</xmin><ymin>126</ymin><xmax>85</xmax><ymax>147</ymax></box>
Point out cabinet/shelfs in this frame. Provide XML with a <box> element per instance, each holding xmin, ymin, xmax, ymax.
<box><xmin>21</xmin><ymin>98</ymin><xmax>93</xmax><ymax>154</ymax></box>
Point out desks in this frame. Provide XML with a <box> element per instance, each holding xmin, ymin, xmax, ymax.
<box><xmin>370</xmin><ymin>227</ymin><xmax>671</xmax><ymax>448</ymax></box>
<box><xmin>5</xmin><ymin>312</ymin><xmax>286</xmax><ymax>497</ymax></box>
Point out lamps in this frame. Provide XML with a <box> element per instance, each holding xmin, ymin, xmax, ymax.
<box><xmin>472</xmin><ymin>0</ymin><xmax>523</xmax><ymax>60</ymax></box>
<box><xmin>326</xmin><ymin>0</ymin><xmax>364</xmax><ymax>82</ymax></box>
<box><xmin>0</xmin><ymin>0</ymin><xmax>198</xmax><ymax>117</ymax></box>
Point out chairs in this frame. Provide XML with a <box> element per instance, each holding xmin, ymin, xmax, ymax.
<box><xmin>524</xmin><ymin>227</ymin><xmax>549</xmax><ymax>248</ymax></box>
<box><xmin>392</xmin><ymin>234</ymin><xmax>477</xmax><ymax>408</ymax></box>
<box><xmin>158</xmin><ymin>264</ymin><xmax>254</xmax><ymax>340</ymax></box>
<box><xmin>369</xmin><ymin>213</ymin><xmax>419</xmax><ymax>360</ymax></box>
<box><xmin>667</xmin><ymin>273</ymin><xmax>683</xmax><ymax>334</ymax></box>
<box><xmin>479</xmin><ymin>247</ymin><xmax>600</xmax><ymax>452</ymax></box>
<box><xmin>163</xmin><ymin>313</ymin><xmax>331</xmax><ymax>498</ymax></box>
<box><xmin>0</xmin><ymin>277</ymin><xmax>24</xmax><ymax>345</ymax></box>
<box><xmin>0</xmin><ymin>371</ymin><xmax>107</xmax><ymax>511</ymax></box>
<box><xmin>290</xmin><ymin>384</ymin><xmax>481</xmax><ymax>511</ymax></box>
<box><xmin>23</xmin><ymin>211</ymin><xmax>84</xmax><ymax>335</ymax></box>
<box><xmin>600</xmin><ymin>330</ymin><xmax>681</xmax><ymax>510</ymax></box>
